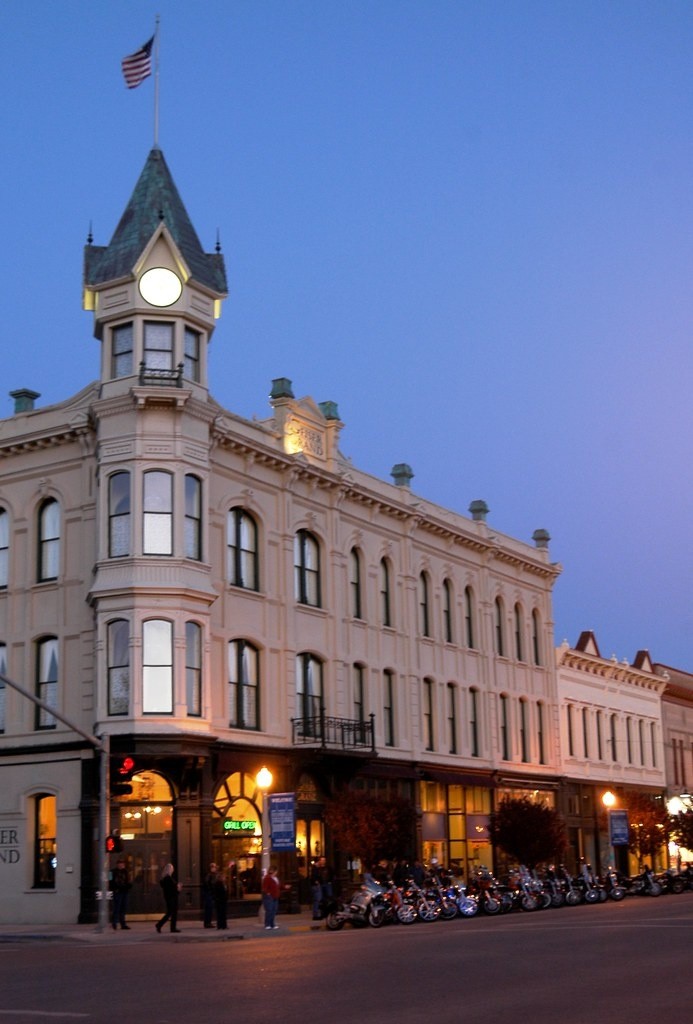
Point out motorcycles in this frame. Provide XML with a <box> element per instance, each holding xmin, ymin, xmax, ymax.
<box><xmin>327</xmin><ymin>858</ymin><xmax>693</xmax><ymax>931</ymax></box>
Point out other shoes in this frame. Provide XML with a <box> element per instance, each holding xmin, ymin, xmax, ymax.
<box><xmin>111</xmin><ymin>922</ymin><xmax>117</xmax><ymax>930</ymax></box>
<box><xmin>121</xmin><ymin>926</ymin><xmax>131</xmax><ymax>930</ymax></box>
<box><xmin>266</xmin><ymin>926</ymin><xmax>280</xmax><ymax>930</ymax></box>
<box><xmin>171</xmin><ymin>928</ymin><xmax>181</xmax><ymax>933</ymax></box>
<box><xmin>216</xmin><ymin>926</ymin><xmax>229</xmax><ymax>930</ymax></box>
<box><xmin>204</xmin><ymin>923</ymin><xmax>215</xmax><ymax>928</ymax></box>
<box><xmin>156</xmin><ymin>923</ymin><xmax>161</xmax><ymax>934</ymax></box>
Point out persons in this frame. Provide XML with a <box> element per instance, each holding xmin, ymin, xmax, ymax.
<box><xmin>110</xmin><ymin>859</ymin><xmax>132</xmax><ymax>929</ymax></box>
<box><xmin>261</xmin><ymin>865</ymin><xmax>293</xmax><ymax>929</ymax></box>
<box><xmin>311</xmin><ymin>856</ymin><xmax>322</xmax><ymax>921</ymax></box>
<box><xmin>320</xmin><ymin>856</ymin><xmax>333</xmax><ymax>899</ymax></box>
<box><xmin>201</xmin><ymin>863</ymin><xmax>219</xmax><ymax>929</ymax></box>
<box><xmin>155</xmin><ymin>863</ymin><xmax>185</xmax><ymax>933</ymax></box>
<box><xmin>214</xmin><ymin>871</ymin><xmax>231</xmax><ymax>930</ymax></box>
<box><xmin>367</xmin><ymin>854</ymin><xmax>465</xmax><ymax>892</ymax></box>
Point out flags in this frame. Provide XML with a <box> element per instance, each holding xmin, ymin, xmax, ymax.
<box><xmin>120</xmin><ymin>34</ymin><xmax>155</xmax><ymax>89</ymax></box>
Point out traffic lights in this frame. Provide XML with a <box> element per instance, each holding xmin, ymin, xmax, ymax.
<box><xmin>109</xmin><ymin>752</ymin><xmax>134</xmax><ymax>798</ymax></box>
<box><xmin>107</xmin><ymin>837</ymin><xmax>123</xmax><ymax>854</ymax></box>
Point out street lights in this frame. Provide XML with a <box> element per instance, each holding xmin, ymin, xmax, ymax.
<box><xmin>256</xmin><ymin>765</ymin><xmax>273</xmax><ymax>926</ymax></box>
<box><xmin>603</xmin><ymin>791</ymin><xmax>616</xmax><ymax>870</ymax></box>
